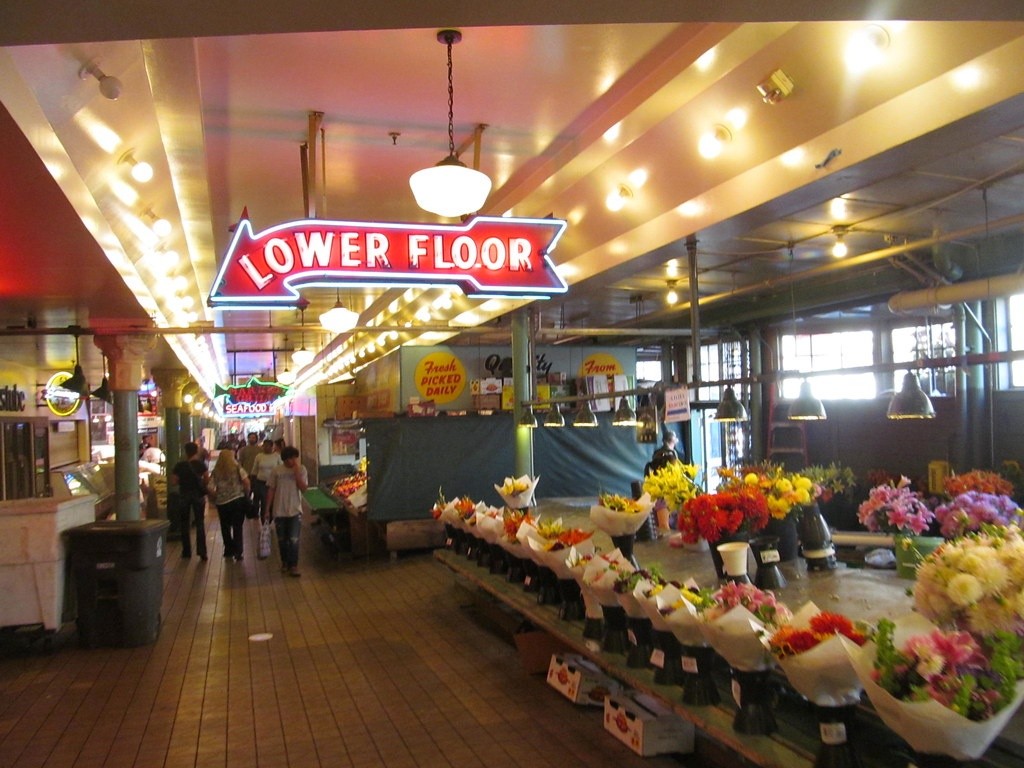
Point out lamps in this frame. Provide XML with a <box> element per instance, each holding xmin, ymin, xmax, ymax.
<box><xmin>272</xmin><ymin>31</ymin><xmax>492</xmax><ymax>386</ymax></box>
<box><xmin>518</xmin><ymin>211</ymin><xmax>937</xmax><ymax>429</ymax></box>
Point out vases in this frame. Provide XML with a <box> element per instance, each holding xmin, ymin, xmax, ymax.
<box><xmin>650</xmin><ymin>630</ymin><xmax>681</xmax><ymax>685</ymax></box>
<box><xmin>600</xmin><ymin>606</ymin><xmax>627</xmax><ymax>652</ymax></box>
<box><xmin>537</xmin><ymin>567</ymin><xmax>559</xmax><ymax>604</ymax></box>
<box><xmin>522</xmin><ymin>559</ymin><xmax>539</xmax><ymax>590</ymax></box>
<box><xmin>812</xmin><ymin>696</ymin><xmax>870</xmax><ymax>768</ymax></box>
<box><xmin>761</xmin><ymin>514</ymin><xmax>799</xmax><ymax>562</ymax></box>
<box><xmin>627</xmin><ymin>618</ymin><xmax>651</xmax><ymax>668</ymax></box>
<box><xmin>730</xmin><ymin>668</ymin><xmax>779</xmax><ymax>735</ymax></box>
<box><xmin>582</xmin><ymin>616</ymin><xmax>604</xmax><ymax>640</ymax></box>
<box><xmin>514</xmin><ymin>507</ymin><xmax>528</xmax><ymax>517</ymax></box>
<box><xmin>709</xmin><ymin>528</ymin><xmax>750</xmax><ymax>581</ymax></box>
<box><xmin>556</xmin><ymin>579</ymin><xmax>584</xmax><ymax>621</ymax></box>
<box><xmin>682</xmin><ymin>645</ymin><xmax>720</xmax><ymax>705</ymax></box>
<box><xmin>612</xmin><ymin>535</ymin><xmax>641</xmax><ymax>573</ymax></box>
<box><xmin>804</xmin><ymin>501</ymin><xmax>831</xmax><ymax>551</ymax></box>
<box><xmin>749</xmin><ymin>537</ymin><xmax>789</xmax><ymax>590</ymax></box>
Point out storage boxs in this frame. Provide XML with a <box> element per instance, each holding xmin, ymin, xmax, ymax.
<box><xmin>546</xmin><ymin>652</ymin><xmax>622</xmax><ymax>705</ymax></box>
<box><xmin>470</xmin><ymin>373</ymin><xmax>569</xmax><ymax>410</ymax></box>
<box><xmin>604</xmin><ymin>689</ymin><xmax>695</xmax><ymax>757</ymax></box>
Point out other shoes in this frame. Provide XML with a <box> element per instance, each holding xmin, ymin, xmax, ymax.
<box><xmin>234</xmin><ymin>551</ymin><xmax>242</xmax><ymax>558</ymax></box>
<box><xmin>182</xmin><ymin>549</ymin><xmax>192</xmax><ymax>557</ymax></box>
<box><xmin>290</xmin><ymin>565</ymin><xmax>301</xmax><ymax>577</ymax></box>
<box><xmin>224</xmin><ymin>548</ymin><xmax>233</xmax><ymax>556</ymax></box>
<box><xmin>198</xmin><ymin>550</ymin><xmax>208</xmax><ymax>561</ymax></box>
<box><xmin>281</xmin><ymin>561</ymin><xmax>288</xmax><ymax>573</ymax></box>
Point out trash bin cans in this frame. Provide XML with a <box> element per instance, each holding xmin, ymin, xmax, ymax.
<box><xmin>61</xmin><ymin>518</ymin><xmax>171</xmax><ymax>650</ymax></box>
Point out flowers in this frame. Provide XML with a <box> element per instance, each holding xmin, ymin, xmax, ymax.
<box><xmin>427</xmin><ymin>461</ymin><xmax>1024</xmax><ymax>757</ymax></box>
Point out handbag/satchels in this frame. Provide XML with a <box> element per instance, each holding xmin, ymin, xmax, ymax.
<box><xmin>258</xmin><ymin>520</ymin><xmax>270</xmax><ymax>557</ymax></box>
<box><xmin>199</xmin><ymin>480</ymin><xmax>208</xmax><ymax>496</ymax></box>
<box><xmin>230</xmin><ymin>497</ymin><xmax>251</xmax><ymax>516</ymax></box>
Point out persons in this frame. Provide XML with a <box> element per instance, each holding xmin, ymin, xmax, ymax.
<box><xmin>251</xmin><ymin>439</ymin><xmax>284</xmax><ymax>526</ymax></box>
<box><xmin>172</xmin><ymin>443</ymin><xmax>209</xmax><ymax>560</ymax></box>
<box><xmin>142</xmin><ymin>435</ymin><xmax>151</xmax><ymax>448</ymax></box>
<box><xmin>217</xmin><ymin>433</ymin><xmax>265</xmax><ymax>460</ymax></box>
<box><xmin>264</xmin><ymin>447</ymin><xmax>308</xmax><ymax>577</ymax></box>
<box><xmin>207</xmin><ymin>450</ymin><xmax>250</xmax><ymax>559</ymax></box>
<box><xmin>238</xmin><ymin>433</ymin><xmax>264</xmax><ymax>520</ymax></box>
<box><xmin>139</xmin><ymin>443</ymin><xmax>147</xmax><ymax>459</ymax></box>
